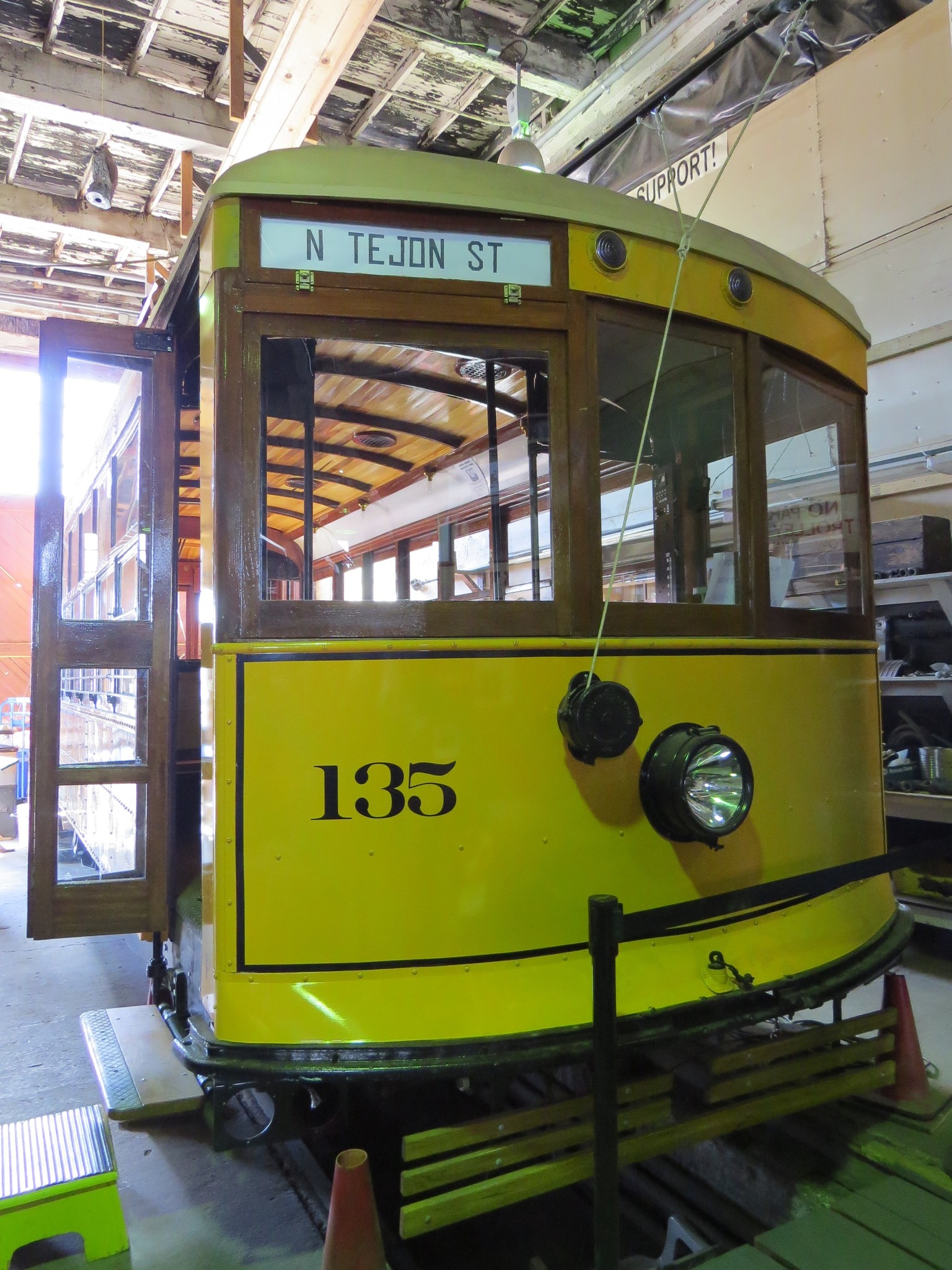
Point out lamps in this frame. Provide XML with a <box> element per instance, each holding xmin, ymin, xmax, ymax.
<box><xmin>497</xmin><ymin>63</ymin><xmax>546</xmax><ymax>173</ymax></box>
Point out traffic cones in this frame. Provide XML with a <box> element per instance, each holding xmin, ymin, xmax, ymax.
<box><xmin>318</xmin><ymin>1149</ymin><xmax>393</xmax><ymax>1270</ymax></box>
<box><xmin>850</xmin><ymin>973</ymin><xmax>952</xmax><ymax>1121</ymax></box>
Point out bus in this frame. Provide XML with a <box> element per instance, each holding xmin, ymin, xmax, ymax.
<box><xmin>21</xmin><ymin>140</ymin><xmax>916</xmax><ymax>1127</ymax></box>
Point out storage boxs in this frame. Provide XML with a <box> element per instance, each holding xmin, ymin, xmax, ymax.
<box><xmin>785</xmin><ymin>514</ymin><xmax>952</xmax><ymax>574</ymax></box>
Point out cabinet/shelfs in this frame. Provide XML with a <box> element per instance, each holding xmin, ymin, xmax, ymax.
<box><xmin>787</xmin><ymin>570</ymin><xmax>952</xmax><ymax>932</ymax></box>
